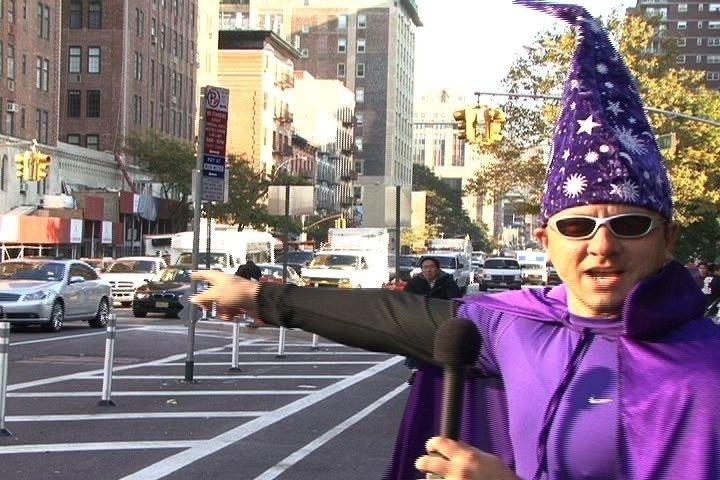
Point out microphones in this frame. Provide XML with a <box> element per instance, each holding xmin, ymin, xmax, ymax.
<box><xmin>432</xmin><ymin>316</ymin><xmax>482</xmax><ymax>451</ymax></box>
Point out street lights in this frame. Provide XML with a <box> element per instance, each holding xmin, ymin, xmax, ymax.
<box><xmin>350</xmin><ymin>181</ymin><xmax>381</xmax><ymax>226</ymax></box>
<box><xmin>269</xmin><ymin>155</ymin><xmax>310</xmax><ymax>181</ymax></box>
<box><xmin>426</xmin><ymin>206</ymin><xmax>453</xmax><ymax>221</ymax></box>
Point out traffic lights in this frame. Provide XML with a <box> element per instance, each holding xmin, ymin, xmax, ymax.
<box><xmin>454</xmin><ymin>108</ymin><xmax>477</xmax><ymax>144</ymax></box>
<box><xmin>335</xmin><ymin>219</ymin><xmax>341</xmax><ymax>228</ymax></box>
<box><xmin>488</xmin><ymin>108</ymin><xmax>505</xmax><ymax>144</ymax></box>
<box><xmin>342</xmin><ymin>218</ymin><xmax>347</xmax><ymax>229</ymax></box>
<box><xmin>35</xmin><ymin>153</ymin><xmax>50</xmax><ymax>181</ymax></box>
<box><xmin>15</xmin><ymin>151</ymin><xmax>31</xmax><ymax>182</ymax></box>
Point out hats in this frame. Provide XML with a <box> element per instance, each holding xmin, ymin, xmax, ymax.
<box><xmin>510</xmin><ymin>0</ymin><xmax>676</xmax><ymax>227</ymax></box>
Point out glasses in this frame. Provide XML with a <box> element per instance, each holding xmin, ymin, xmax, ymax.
<box><xmin>543</xmin><ymin>211</ymin><xmax>666</xmax><ymax>242</ymax></box>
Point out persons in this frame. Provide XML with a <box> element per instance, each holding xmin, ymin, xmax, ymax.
<box><xmin>402</xmin><ymin>257</ymin><xmax>463</xmax><ymax>385</ymax></box>
<box><xmin>189</xmin><ymin>1</ymin><xmax>720</xmax><ymax>480</ymax></box>
<box><xmin>685</xmin><ymin>259</ymin><xmax>720</xmax><ymax>327</ymax></box>
<box><xmin>236</xmin><ymin>252</ymin><xmax>262</xmax><ymax>281</ymax></box>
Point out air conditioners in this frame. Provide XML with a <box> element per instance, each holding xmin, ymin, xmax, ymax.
<box><xmin>7</xmin><ymin>103</ymin><xmax>19</xmax><ymax>113</ymax></box>
